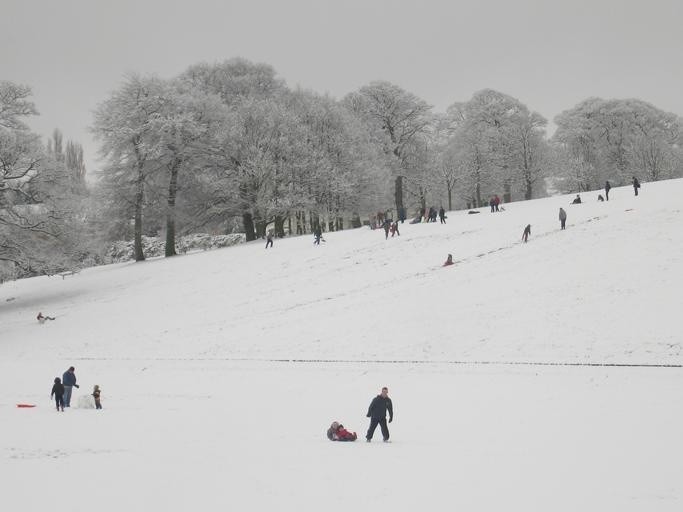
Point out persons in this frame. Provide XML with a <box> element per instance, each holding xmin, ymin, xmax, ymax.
<box><xmin>50</xmin><ymin>367</ymin><xmax>79</xmax><ymax>411</ymax></box>
<box><xmin>37</xmin><ymin>311</ymin><xmax>55</xmax><ymax>324</ymax></box>
<box><xmin>572</xmin><ymin>193</ymin><xmax>581</xmax><ymax>203</ymax></box>
<box><xmin>630</xmin><ymin>176</ymin><xmax>641</xmax><ymax>197</ymax></box>
<box><xmin>314</xmin><ymin>225</ymin><xmax>320</xmax><ymax>245</ymax></box>
<box><xmin>467</xmin><ymin>192</ymin><xmax>500</xmax><ymax>213</ymax></box>
<box><xmin>368</xmin><ymin>207</ymin><xmax>399</xmax><ymax>239</ymax></box>
<box><xmin>522</xmin><ymin>224</ymin><xmax>530</xmax><ymax>243</ymax></box>
<box><xmin>366</xmin><ymin>387</ymin><xmax>394</xmax><ymax>444</ymax></box>
<box><xmin>559</xmin><ymin>208</ymin><xmax>567</xmax><ymax>230</ymax></box>
<box><xmin>265</xmin><ymin>233</ymin><xmax>274</xmax><ymax>248</ymax></box>
<box><xmin>444</xmin><ymin>254</ymin><xmax>454</xmax><ymax>266</ymax></box>
<box><xmin>327</xmin><ymin>422</ymin><xmax>358</xmax><ymax>441</ymax></box>
<box><xmin>419</xmin><ymin>205</ymin><xmax>446</xmax><ymax>225</ymax></box>
<box><xmin>91</xmin><ymin>384</ymin><xmax>102</xmax><ymax>410</ymax></box>
<box><xmin>599</xmin><ymin>180</ymin><xmax>610</xmax><ymax>201</ymax></box>
